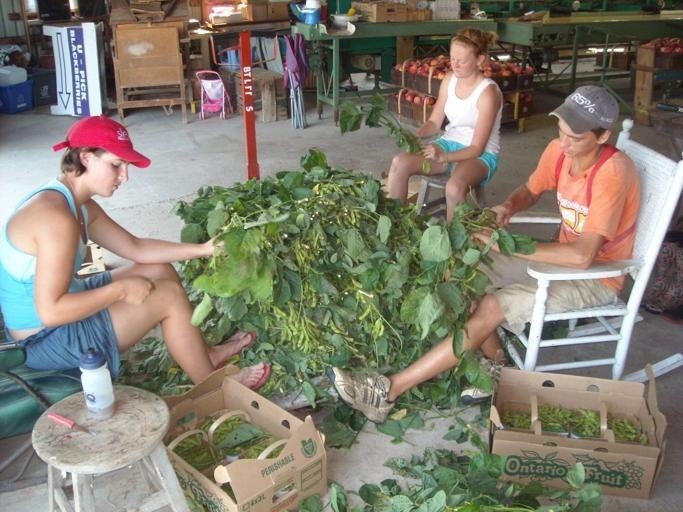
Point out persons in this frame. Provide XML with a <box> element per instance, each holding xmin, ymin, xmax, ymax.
<box><xmin>324</xmin><ymin>85</ymin><xmax>639</xmax><ymax>425</ymax></box>
<box><xmin>0</xmin><ymin>116</ymin><xmax>272</xmax><ymax>397</ymax></box>
<box><xmin>385</xmin><ymin>29</ymin><xmax>502</xmax><ymax>224</ymax></box>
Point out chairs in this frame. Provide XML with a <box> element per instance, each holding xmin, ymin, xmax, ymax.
<box><xmin>489</xmin><ymin>119</ymin><xmax>682</xmax><ymax>382</ymax></box>
<box><xmin>0</xmin><ymin>307</ymin><xmax>87</xmax><ymax>495</ymax></box>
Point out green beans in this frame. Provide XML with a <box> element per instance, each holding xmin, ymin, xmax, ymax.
<box><xmin>289</xmin><ymin>236</ymin><xmax>320</xmax><ymax>308</ymax></box>
<box><xmin>304</xmin><ymin>182</ymin><xmax>362</xmax><ymax>228</ymax></box>
<box><xmin>348</xmin><ymin>276</ymin><xmax>385</xmax><ymax>338</ymax></box>
<box><xmin>257</xmin><ymin>299</ymin><xmax>319</xmax><ymax>354</ymax></box>
<box><xmin>160</xmin><ymin>409</ymin><xmax>290</xmax><ymax>505</ymax></box>
<box><xmin>496</xmin><ymin>401</ymin><xmax>652</xmax><ymax>448</ymax></box>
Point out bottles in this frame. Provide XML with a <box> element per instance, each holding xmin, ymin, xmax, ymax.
<box><xmin>78</xmin><ymin>347</ymin><xmax>117</xmax><ymax>419</ymax></box>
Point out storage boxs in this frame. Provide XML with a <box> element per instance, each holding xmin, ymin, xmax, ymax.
<box><xmin>236</xmin><ymin>2</ymin><xmax>268</xmax><ymax>21</ymax></box>
<box><xmin>39</xmin><ymin>49</ymin><xmax>55</xmax><ymax>69</ymax></box>
<box><xmin>268</xmin><ymin>2</ymin><xmax>289</xmax><ymax>22</ymax></box>
<box><xmin>486</xmin><ymin>367</ymin><xmax>666</xmax><ymax>501</ymax></box>
<box><xmin>75</xmin><ymin>241</ymin><xmax>107</xmax><ymax>279</ymax></box>
<box><xmin>376</xmin><ymin>182</ymin><xmax>418</xmax><ymax>206</ymax></box>
<box><xmin>157</xmin><ymin>362</ymin><xmax>330</xmax><ymax>509</ymax></box>
<box><xmin>26</xmin><ymin>71</ymin><xmax>56</xmax><ymax>108</ymax></box>
<box><xmin>0</xmin><ymin>77</ymin><xmax>33</xmax><ymax>115</ymax></box>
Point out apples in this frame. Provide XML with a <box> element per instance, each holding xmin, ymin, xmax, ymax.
<box><xmin>501</xmin><ymin>89</ymin><xmax>536</xmax><ymax>106</ymax></box>
<box><xmin>394</xmin><ymin>54</ymin><xmax>535</xmax><ymax>80</ymax></box>
<box><xmin>639</xmin><ymin>37</ymin><xmax>683</xmax><ymax>55</ymax></box>
<box><xmin>394</xmin><ymin>86</ymin><xmax>437</xmax><ymax>108</ymax></box>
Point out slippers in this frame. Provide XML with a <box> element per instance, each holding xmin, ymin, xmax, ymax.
<box><xmin>239</xmin><ymin>363</ymin><xmax>271</xmax><ymax>391</ymax></box>
<box><xmin>214</xmin><ymin>329</ymin><xmax>256</xmax><ymax>371</ymax></box>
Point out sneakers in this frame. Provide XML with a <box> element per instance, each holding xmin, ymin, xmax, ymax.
<box><xmin>326</xmin><ymin>366</ymin><xmax>396</xmax><ymax>425</ymax></box>
<box><xmin>460</xmin><ymin>355</ymin><xmax>505</xmax><ymax>405</ymax></box>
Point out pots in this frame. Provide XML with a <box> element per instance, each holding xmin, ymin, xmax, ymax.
<box><xmin>330</xmin><ymin>14</ymin><xmax>362</xmax><ymax>28</ymax></box>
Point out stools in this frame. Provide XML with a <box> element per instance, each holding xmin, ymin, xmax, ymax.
<box><xmin>31</xmin><ymin>384</ymin><xmax>187</xmax><ymax>512</ymax></box>
<box><xmin>420</xmin><ymin>174</ymin><xmax>482</xmax><ymax>217</ymax></box>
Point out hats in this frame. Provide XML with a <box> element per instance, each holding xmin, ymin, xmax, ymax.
<box><xmin>547</xmin><ymin>85</ymin><xmax>620</xmax><ymax>135</ymax></box>
<box><xmin>52</xmin><ymin>115</ymin><xmax>151</xmax><ymax>168</ymax></box>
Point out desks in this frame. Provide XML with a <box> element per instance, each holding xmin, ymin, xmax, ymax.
<box><xmin>184</xmin><ymin>22</ymin><xmax>292</xmax><ymax>111</ymax></box>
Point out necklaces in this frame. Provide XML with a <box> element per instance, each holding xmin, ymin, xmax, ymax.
<box><xmin>65</xmin><ymin>178</ymin><xmax>84</xmax><ymax>225</ymax></box>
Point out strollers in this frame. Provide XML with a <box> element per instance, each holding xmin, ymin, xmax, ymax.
<box><xmin>195</xmin><ymin>68</ymin><xmax>234</xmax><ymax>120</ymax></box>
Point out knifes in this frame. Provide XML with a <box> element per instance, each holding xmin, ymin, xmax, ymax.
<box><xmin>47</xmin><ymin>411</ymin><xmax>92</xmax><ymax>434</ymax></box>
